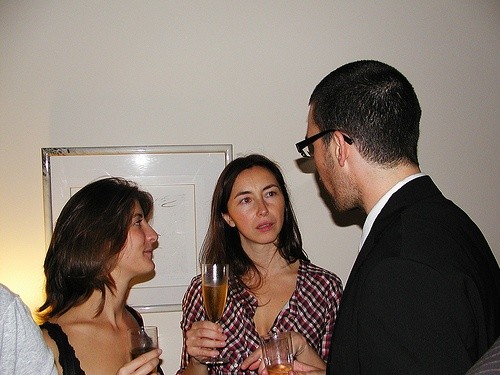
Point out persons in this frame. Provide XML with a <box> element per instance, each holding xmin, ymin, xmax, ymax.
<box><xmin>174</xmin><ymin>154</ymin><xmax>343</xmax><ymax>375</ymax></box>
<box><xmin>36</xmin><ymin>176</ymin><xmax>165</xmax><ymax>375</ymax></box>
<box><xmin>0</xmin><ymin>283</ymin><xmax>60</xmax><ymax>375</ymax></box>
<box><xmin>261</xmin><ymin>60</ymin><xmax>500</xmax><ymax>375</ymax></box>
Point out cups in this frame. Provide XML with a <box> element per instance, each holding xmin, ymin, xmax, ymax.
<box><xmin>259</xmin><ymin>332</ymin><xmax>294</xmax><ymax>374</ymax></box>
<box><xmin>126</xmin><ymin>326</ymin><xmax>159</xmax><ymax>375</ymax></box>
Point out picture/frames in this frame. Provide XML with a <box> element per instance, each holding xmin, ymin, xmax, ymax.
<box><xmin>40</xmin><ymin>144</ymin><xmax>232</xmax><ymax>313</ymax></box>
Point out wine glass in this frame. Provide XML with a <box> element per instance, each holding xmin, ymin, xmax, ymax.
<box><xmin>199</xmin><ymin>263</ymin><xmax>230</xmax><ymax>365</ymax></box>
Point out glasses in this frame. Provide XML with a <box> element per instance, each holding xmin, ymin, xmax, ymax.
<box><xmin>295</xmin><ymin>128</ymin><xmax>354</xmax><ymax>158</ymax></box>
